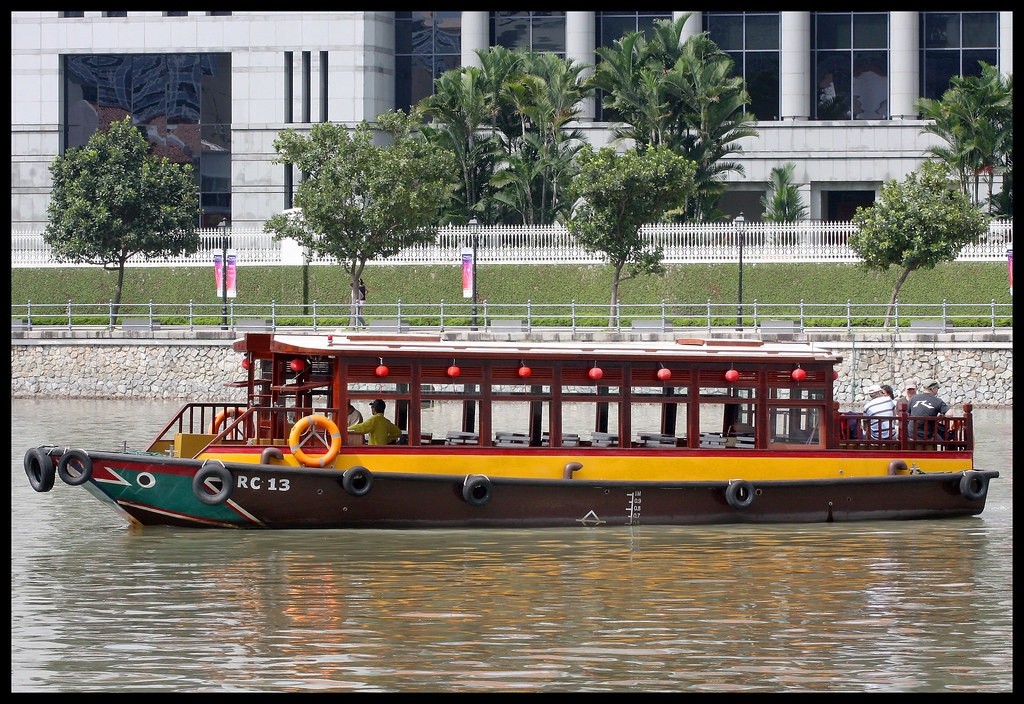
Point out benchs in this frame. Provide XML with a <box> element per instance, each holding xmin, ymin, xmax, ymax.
<box><xmin>401</xmin><ymin>431</ymin><xmax>774</xmax><ymax>449</ymax></box>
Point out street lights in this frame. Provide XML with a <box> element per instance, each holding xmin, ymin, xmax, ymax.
<box><xmin>468</xmin><ymin>215</ymin><xmax>481</xmax><ymax>332</ymax></box>
<box><xmin>734</xmin><ymin>212</ymin><xmax>747</xmax><ymax>332</ymax></box>
<box><xmin>216</xmin><ymin>217</ymin><xmax>233</xmax><ymax>331</ymax></box>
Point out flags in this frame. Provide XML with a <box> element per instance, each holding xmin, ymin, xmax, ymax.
<box><xmin>462</xmin><ymin>254</ymin><xmax>473</xmax><ymax>298</ymax></box>
<box><xmin>1007</xmin><ymin>243</ymin><xmax>1013</xmax><ymax>296</ymax></box>
<box><xmin>214</xmin><ymin>255</ymin><xmax>223</xmax><ymax>297</ymax></box>
<box><xmin>226</xmin><ymin>255</ymin><xmax>237</xmax><ymax>298</ymax></box>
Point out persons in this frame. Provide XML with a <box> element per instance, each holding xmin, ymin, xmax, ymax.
<box><xmin>348</xmin><ymin>404</ymin><xmax>363</xmax><ymax>427</ymax></box>
<box><xmin>346</xmin><ymin>278</ymin><xmax>369</xmax><ymax>330</ymax></box>
<box><xmin>347</xmin><ymin>399</ymin><xmax>402</xmax><ymax>445</ymax></box>
<box><xmin>863</xmin><ymin>380</ymin><xmax>973</xmax><ymax>440</ymax></box>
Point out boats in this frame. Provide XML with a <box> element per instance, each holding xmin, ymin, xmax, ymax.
<box><xmin>23</xmin><ymin>332</ymin><xmax>997</xmax><ymax>531</ymax></box>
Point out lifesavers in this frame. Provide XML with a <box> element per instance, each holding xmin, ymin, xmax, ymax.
<box><xmin>462</xmin><ymin>475</ymin><xmax>493</xmax><ymax>507</ymax></box>
<box><xmin>58</xmin><ymin>449</ymin><xmax>93</xmax><ymax>486</ymax></box>
<box><xmin>192</xmin><ymin>464</ymin><xmax>233</xmax><ymax>506</ymax></box>
<box><xmin>725</xmin><ymin>480</ymin><xmax>756</xmax><ymax>509</ymax></box>
<box><xmin>342</xmin><ymin>465</ymin><xmax>374</xmax><ymax>497</ymax></box>
<box><xmin>209</xmin><ymin>408</ymin><xmax>256</xmax><ymax>438</ymax></box>
<box><xmin>289</xmin><ymin>414</ymin><xmax>342</xmax><ymax>468</ymax></box>
<box><xmin>23</xmin><ymin>446</ymin><xmax>56</xmax><ymax>493</ymax></box>
<box><xmin>959</xmin><ymin>471</ymin><xmax>988</xmax><ymax>501</ymax></box>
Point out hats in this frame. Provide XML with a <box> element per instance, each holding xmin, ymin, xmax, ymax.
<box><xmin>923</xmin><ymin>380</ymin><xmax>941</xmax><ymax>388</ymax></box>
<box><xmin>864</xmin><ymin>385</ymin><xmax>883</xmax><ymax>393</ymax></box>
<box><xmin>369</xmin><ymin>399</ymin><xmax>385</xmax><ymax>409</ymax></box>
<box><xmin>906</xmin><ymin>385</ymin><xmax>916</xmax><ymax>389</ymax></box>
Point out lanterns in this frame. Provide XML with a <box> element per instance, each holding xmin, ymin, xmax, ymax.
<box><xmin>519</xmin><ymin>366</ymin><xmax>530</xmax><ymax>377</ymax></box>
<box><xmin>589</xmin><ymin>368</ymin><xmax>603</xmax><ymax>380</ymax></box>
<box><xmin>792</xmin><ymin>369</ymin><xmax>806</xmax><ymax>381</ymax></box>
<box><xmin>448</xmin><ymin>365</ymin><xmax>460</xmax><ymax>377</ymax></box>
<box><xmin>376</xmin><ymin>366</ymin><xmax>388</xmax><ymax>377</ymax></box>
<box><xmin>290</xmin><ymin>360</ymin><xmax>304</xmax><ymax>371</ymax></box>
<box><xmin>242</xmin><ymin>357</ymin><xmax>248</xmax><ymax>370</ymax></box>
<box><xmin>657</xmin><ymin>369</ymin><xmax>671</xmax><ymax>380</ymax></box>
<box><xmin>833</xmin><ymin>369</ymin><xmax>838</xmax><ymax>381</ymax></box>
<box><xmin>725</xmin><ymin>370</ymin><xmax>739</xmax><ymax>382</ymax></box>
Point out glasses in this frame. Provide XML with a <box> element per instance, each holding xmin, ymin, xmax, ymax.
<box><xmin>882</xmin><ymin>392</ymin><xmax>885</xmax><ymax>394</ymax></box>
